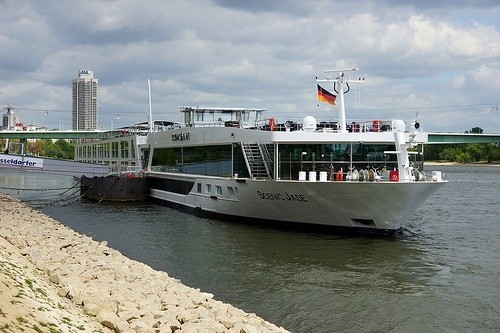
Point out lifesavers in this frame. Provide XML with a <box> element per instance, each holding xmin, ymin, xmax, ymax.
<box><xmin>373</xmin><ymin>120</ymin><xmax>380</xmax><ymax>132</ymax></box>
<box><xmin>269</xmin><ymin>117</ymin><xmax>276</xmax><ymax>130</ymax></box>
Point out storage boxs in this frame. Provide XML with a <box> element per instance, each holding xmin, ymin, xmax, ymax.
<box><xmin>389</xmin><ymin>170</ymin><xmax>399</xmax><ymax>182</ymax></box>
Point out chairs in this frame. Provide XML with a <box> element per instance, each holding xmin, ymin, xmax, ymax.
<box><xmin>412</xmin><ymin>168</ymin><xmax>427</xmax><ymax>181</ymax></box>
<box><xmin>345</xmin><ymin>169</ymin><xmax>390</xmax><ymax>182</ymax></box>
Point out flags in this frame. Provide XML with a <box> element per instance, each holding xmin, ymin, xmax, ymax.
<box><xmin>317</xmin><ymin>84</ymin><xmax>336</xmax><ymax>105</ymax></box>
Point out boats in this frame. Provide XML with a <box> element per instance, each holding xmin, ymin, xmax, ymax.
<box><xmin>77</xmin><ymin>172</ymin><xmax>152</xmax><ymax>204</ymax></box>
<box><xmin>134</xmin><ymin>66</ymin><xmax>453</xmax><ymax>243</ymax></box>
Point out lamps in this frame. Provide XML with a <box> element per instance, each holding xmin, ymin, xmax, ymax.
<box><xmin>45</xmin><ymin>110</ymin><xmax>48</xmax><ymax>116</ymax></box>
<box><xmin>117</xmin><ymin>113</ymin><xmax>120</xmax><ymax>120</ymax></box>
